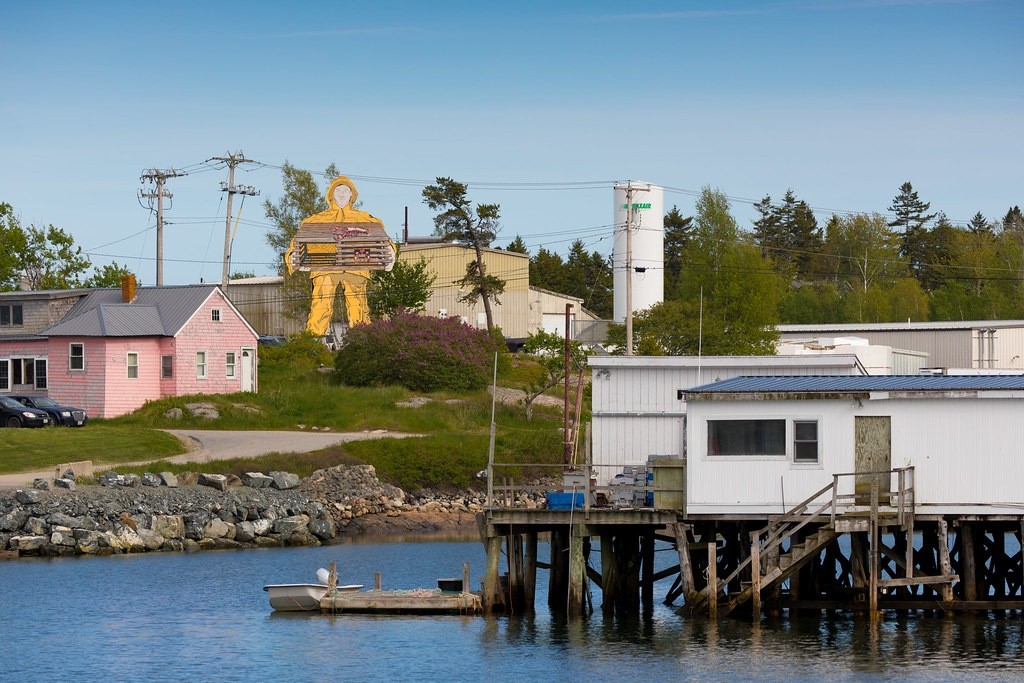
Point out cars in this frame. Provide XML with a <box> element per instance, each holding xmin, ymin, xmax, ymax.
<box><xmin>0</xmin><ymin>396</ymin><xmax>51</xmax><ymax>429</ymax></box>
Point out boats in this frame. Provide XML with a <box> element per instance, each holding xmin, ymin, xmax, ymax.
<box><xmin>263</xmin><ymin>584</ymin><xmax>364</xmax><ymax>611</ymax></box>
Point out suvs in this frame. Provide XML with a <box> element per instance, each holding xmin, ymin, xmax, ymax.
<box><xmin>311</xmin><ymin>335</ymin><xmax>336</xmax><ymax>352</ymax></box>
<box><xmin>5</xmin><ymin>395</ymin><xmax>88</xmax><ymax>427</ymax></box>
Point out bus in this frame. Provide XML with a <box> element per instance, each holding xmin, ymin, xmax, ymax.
<box><xmin>259</xmin><ymin>334</ymin><xmax>288</xmax><ymax>345</ymax></box>
